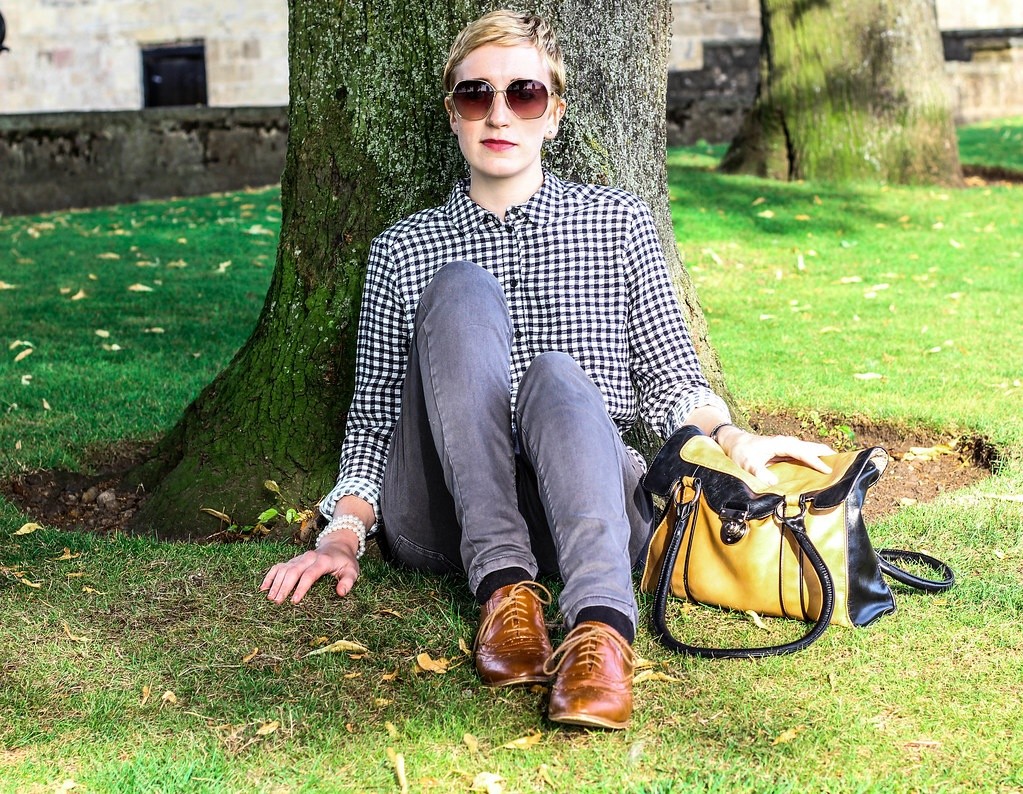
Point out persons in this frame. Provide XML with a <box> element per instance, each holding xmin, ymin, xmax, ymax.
<box><xmin>262</xmin><ymin>8</ymin><xmax>840</xmax><ymax>728</ymax></box>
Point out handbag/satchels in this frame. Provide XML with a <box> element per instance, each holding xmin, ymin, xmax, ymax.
<box><xmin>639</xmin><ymin>424</ymin><xmax>956</xmax><ymax>660</ymax></box>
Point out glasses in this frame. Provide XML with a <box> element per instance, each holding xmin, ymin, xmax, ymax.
<box><xmin>445</xmin><ymin>78</ymin><xmax>556</xmax><ymax>122</ymax></box>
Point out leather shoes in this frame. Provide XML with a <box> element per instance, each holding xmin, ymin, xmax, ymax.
<box><xmin>471</xmin><ymin>580</ymin><xmax>555</xmax><ymax>689</ymax></box>
<box><xmin>542</xmin><ymin>620</ymin><xmax>636</xmax><ymax>730</ymax></box>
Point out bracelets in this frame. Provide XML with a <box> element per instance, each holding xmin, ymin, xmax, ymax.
<box><xmin>316</xmin><ymin>515</ymin><xmax>366</xmax><ymax>560</ymax></box>
<box><xmin>710</xmin><ymin>422</ymin><xmax>734</xmax><ymax>442</ymax></box>
